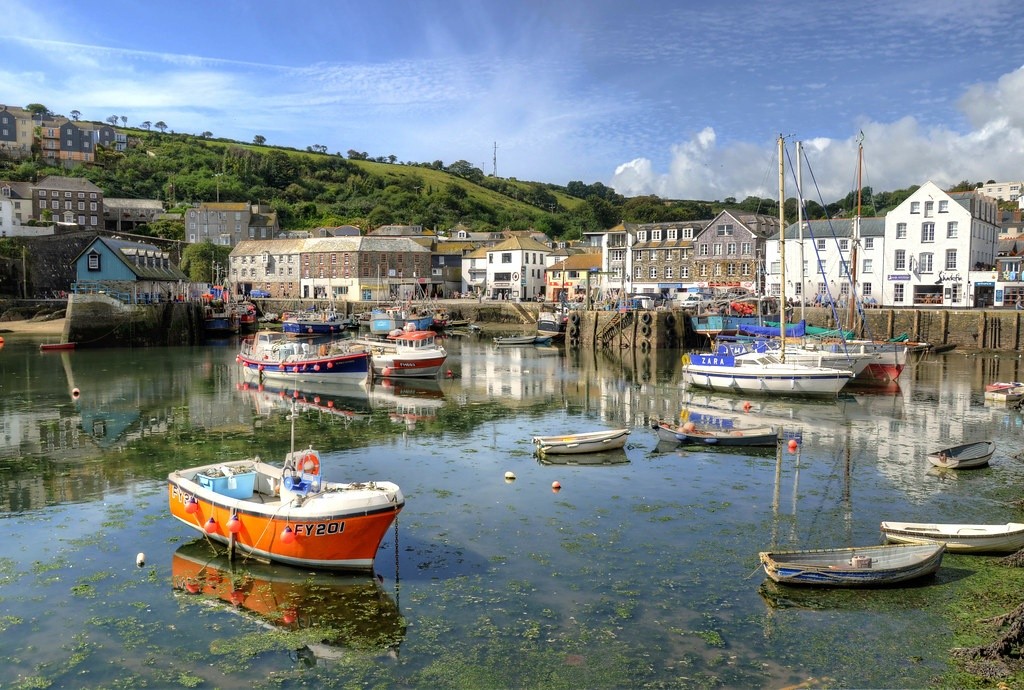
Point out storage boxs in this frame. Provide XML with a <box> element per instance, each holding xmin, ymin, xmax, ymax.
<box><xmin>197</xmin><ymin>471</ymin><xmax>257</xmax><ymax>499</ymax></box>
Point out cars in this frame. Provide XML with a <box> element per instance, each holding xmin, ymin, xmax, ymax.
<box><xmin>249</xmin><ymin>289</ymin><xmax>272</xmax><ymax>299</ymax></box>
<box><xmin>680</xmin><ymin>295</ymin><xmax>705</xmax><ymax>312</ymax></box>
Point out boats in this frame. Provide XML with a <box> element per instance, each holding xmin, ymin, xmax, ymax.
<box><xmin>984</xmin><ymin>380</ymin><xmax>1024</xmax><ymax>404</ymax></box>
<box><xmin>359</xmin><ymin>375</ymin><xmax>448</xmax><ymax>431</ymax></box>
<box><xmin>169</xmin><ymin>534</ymin><xmax>409</xmax><ymax>663</ymax></box>
<box><xmin>653</xmin><ymin>417</ymin><xmax>779</xmax><ymax>447</ymax></box>
<box><xmin>532</xmin><ymin>447</ymin><xmax>633</xmax><ymax>467</ymax></box>
<box><xmin>492</xmin><ymin>334</ymin><xmax>536</xmax><ymax>344</ymax></box>
<box><xmin>236</xmin><ymin>371</ymin><xmax>373</xmax><ymax>429</ymax></box>
<box><xmin>363</xmin><ymin>316</ymin><xmax>448</xmax><ymax>378</ymax></box>
<box><xmin>39</xmin><ymin>341</ymin><xmax>78</xmax><ymax>350</ymax></box>
<box><xmin>167</xmin><ymin>394</ymin><xmax>406</xmax><ymax>574</ymax></box>
<box><xmin>927</xmin><ymin>439</ymin><xmax>996</xmax><ymax>469</ymax></box>
<box><xmin>236</xmin><ymin>329</ymin><xmax>374</xmax><ymax>385</ymax></box>
<box><xmin>531</xmin><ymin>428</ymin><xmax>632</xmax><ymax>454</ymax></box>
<box><xmin>758</xmin><ymin>541</ymin><xmax>947</xmax><ymax>590</ymax></box>
<box><xmin>880</xmin><ymin>520</ymin><xmax>1024</xmax><ymax>555</ymax></box>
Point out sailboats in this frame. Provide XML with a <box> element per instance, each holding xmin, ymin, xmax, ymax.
<box><xmin>681</xmin><ymin>126</ymin><xmax>960</xmax><ymax>401</ymax></box>
<box><xmin>196</xmin><ymin>265</ymin><xmax>472</xmax><ymax>340</ymax></box>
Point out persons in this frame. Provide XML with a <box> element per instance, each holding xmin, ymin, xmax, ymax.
<box><xmin>449</xmin><ymin>290</ymin><xmax>460</xmax><ymax>299</ymax></box>
<box><xmin>704</xmin><ymin>303</ymin><xmax>713</xmax><ymax>313</ymax></box>
<box><xmin>1015</xmin><ymin>294</ymin><xmax>1023</xmax><ymax>309</ymax></box>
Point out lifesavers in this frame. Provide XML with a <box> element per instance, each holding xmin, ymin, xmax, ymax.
<box><xmin>570</xmin><ymin>325</ymin><xmax>579</xmax><ymax>337</ymax></box>
<box><xmin>298</xmin><ymin>453</ymin><xmax>320</xmax><ymax>475</ymax></box>
<box><xmin>665</xmin><ymin>328</ymin><xmax>676</xmax><ymax>348</ymax></box>
<box><xmin>642</xmin><ymin>312</ymin><xmax>651</xmax><ymax>324</ymax></box>
<box><xmin>640</xmin><ymin>339</ymin><xmax>650</xmax><ymax>349</ymax></box>
<box><xmin>707</xmin><ymin>281</ymin><xmax>740</xmax><ymax>286</ymax></box>
<box><xmin>666</xmin><ymin>314</ymin><xmax>676</xmax><ymax>327</ymax></box>
<box><xmin>640</xmin><ymin>325</ymin><xmax>651</xmax><ymax>336</ymax></box>
<box><xmin>569</xmin><ymin>338</ymin><xmax>578</xmax><ymax>349</ymax></box>
<box><xmin>570</xmin><ymin>313</ymin><xmax>580</xmax><ymax>324</ymax></box>
